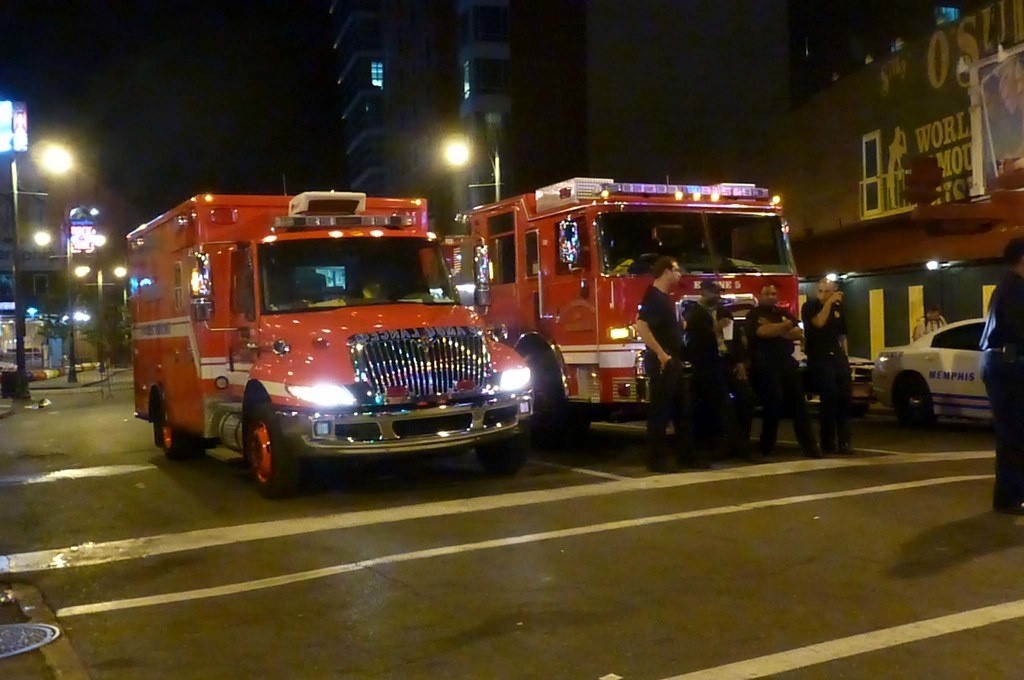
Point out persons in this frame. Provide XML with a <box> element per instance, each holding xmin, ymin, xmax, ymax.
<box><xmin>912</xmin><ymin>306</ymin><xmax>947</xmax><ymax>342</ymax></box>
<box><xmin>681</xmin><ymin>276</ymin><xmax>748</xmax><ymax>459</ymax></box>
<box><xmin>979</xmin><ymin>235</ymin><xmax>1024</xmax><ymax>513</ymax></box>
<box><xmin>740</xmin><ymin>282</ymin><xmax>823</xmax><ymax>460</ymax></box>
<box><xmin>634</xmin><ymin>257</ymin><xmax>709</xmax><ymax>474</ymax></box>
<box><xmin>801</xmin><ymin>281</ymin><xmax>855</xmax><ymax>455</ymax></box>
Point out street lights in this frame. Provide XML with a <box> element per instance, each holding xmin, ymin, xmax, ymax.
<box><xmin>74</xmin><ymin>264</ymin><xmax>127</xmax><ymax>372</ymax></box>
<box><xmin>6</xmin><ymin>148</ymin><xmax>71</xmax><ymax>401</ymax></box>
<box><xmin>444</xmin><ymin>139</ymin><xmax>503</xmax><ymax>205</ymax></box>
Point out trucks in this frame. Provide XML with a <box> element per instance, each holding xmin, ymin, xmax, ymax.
<box><xmin>124</xmin><ymin>190</ymin><xmax>534</xmax><ymax>498</ymax></box>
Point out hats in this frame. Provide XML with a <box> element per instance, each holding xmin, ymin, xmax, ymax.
<box><xmin>701</xmin><ymin>277</ymin><xmax>725</xmax><ymax>294</ymax></box>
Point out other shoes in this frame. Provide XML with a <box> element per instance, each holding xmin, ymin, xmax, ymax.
<box><xmin>822</xmin><ymin>443</ymin><xmax>837</xmax><ymax>454</ymax></box>
<box><xmin>647</xmin><ymin>462</ymin><xmax>671</xmax><ymax>473</ymax></box>
<box><xmin>688</xmin><ymin>460</ymin><xmax>710</xmax><ymax>470</ymax></box>
<box><xmin>759</xmin><ymin>442</ymin><xmax>770</xmax><ymax>456</ymax></box>
<box><xmin>993</xmin><ymin>502</ymin><xmax>1024</xmax><ymax>515</ymax></box>
<box><xmin>839</xmin><ymin>442</ymin><xmax>852</xmax><ymax>453</ymax></box>
<box><xmin>801</xmin><ymin>448</ymin><xmax>821</xmax><ymax>458</ymax></box>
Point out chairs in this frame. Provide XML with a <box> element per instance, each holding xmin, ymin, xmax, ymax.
<box><xmin>264</xmin><ymin>262</ymin><xmax>328</xmax><ymax>302</ymax></box>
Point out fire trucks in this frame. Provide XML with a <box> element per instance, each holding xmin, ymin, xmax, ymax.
<box><xmin>438</xmin><ymin>175</ymin><xmax>800</xmax><ymax>460</ymax></box>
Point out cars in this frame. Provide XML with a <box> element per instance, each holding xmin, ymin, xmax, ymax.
<box><xmin>796</xmin><ymin>354</ymin><xmax>872</xmax><ymax>415</ymax></box>
<box><xmin>868</xmin><ymin>318</ymin><xmax>990</xmax><ymax>429</ymax></box>
